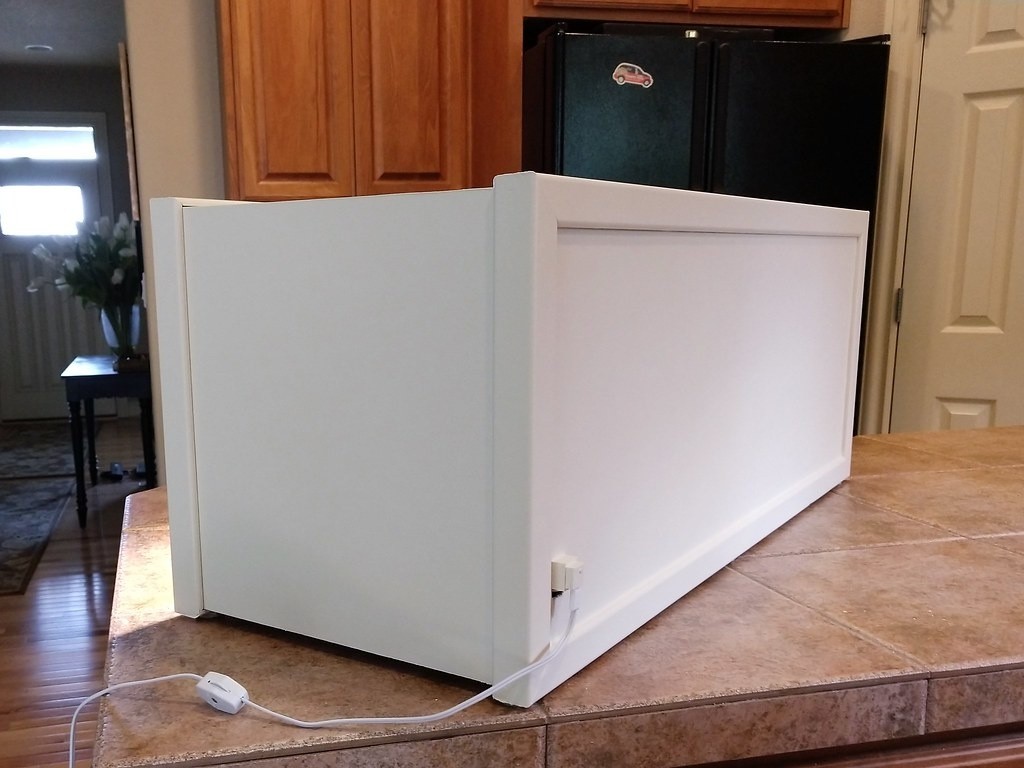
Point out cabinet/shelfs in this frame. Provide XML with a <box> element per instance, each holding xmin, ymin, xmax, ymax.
<box><xmin>211</xmin><ymin>0</ymin><xmax>523</xmax><ymax>203</ymax></box>
<box><xmin>523</xmin><ymin>0</ymin><xmax>851</xmax><ymax>28</ymax></box>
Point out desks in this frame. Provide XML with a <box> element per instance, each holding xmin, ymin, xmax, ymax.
<box><xmin>62</xmin><ymin>355</ymin><xmax>158</xmax><ymax>528</ymax></box>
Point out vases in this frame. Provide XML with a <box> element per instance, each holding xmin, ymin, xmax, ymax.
<box><xmin>98</xmin><ymin>302</ymin><xmax>147</xmax><ymax>372</ymax></box>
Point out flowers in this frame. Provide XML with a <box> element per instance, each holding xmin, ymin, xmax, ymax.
<box><xmin>24</xmin><ymin>212</ymin><xmax>142</xmax><ymax>305</ymax></box>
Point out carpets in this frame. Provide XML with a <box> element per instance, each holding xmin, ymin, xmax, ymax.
<box><xmin>0</xmin><ymin>414</ymin><xmax>102</xmax><ymax>598</ymax></box>
